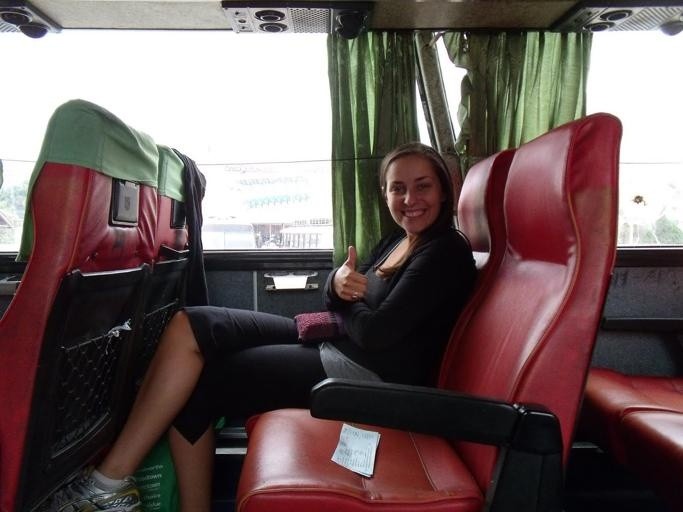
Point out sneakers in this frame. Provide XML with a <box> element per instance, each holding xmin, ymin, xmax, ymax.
<box><xmin>34</xmin><ymin>465</ymin><xmax>143</xmax><ymax>512</ymax></box>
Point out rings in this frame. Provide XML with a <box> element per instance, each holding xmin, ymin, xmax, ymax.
<box><xmin>351</xmin><ymin>291</ymin><xmax>358</xmax><ymax>298</ymax></box>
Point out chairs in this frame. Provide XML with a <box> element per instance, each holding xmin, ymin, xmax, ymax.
<box><xmin>581</xmin><ymin>367</ymin><xmax>682</xmax><ymax>511</ymax></box>
<box><xmin>1</xmin><ymin>98</ymin><xmax>206</xmax><ymax>512</ymax></box>
<box><xmin>234</xmin><ymin>111</ymin><xmax>624</xmax><ymax>511</ymax></box>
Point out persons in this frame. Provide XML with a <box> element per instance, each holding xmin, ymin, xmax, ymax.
<box><xmin>31</xmin><ymin>139</ymin><xmax>478</xmax><ymax>512</ymax></box>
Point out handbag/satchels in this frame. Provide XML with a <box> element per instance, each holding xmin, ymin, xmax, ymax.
<box><xmin>294</xmin><ymin>311</ymin><xmax>346</xmax><ymax>345</ymax></box>
<box><xmin>134</xmin><ymin>416</ymin><xmax>225</xmax><ymax>512</ymax></box>
